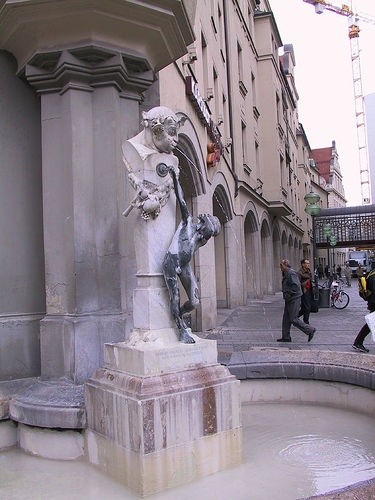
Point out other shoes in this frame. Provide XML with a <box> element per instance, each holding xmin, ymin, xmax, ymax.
<box><xmin>308</xmin><ymin>328</ymin><xmax>315</xmax><ymax>341</ymax></box>
<box><xmin>351</xmin><ymin>343</ymin><xmax>369</xmax><ymax>352</ymax></box>
<box><xmin>277</xmin><ymin>336</ymin><xmax>291</xmax><ymax>342</ymax></box>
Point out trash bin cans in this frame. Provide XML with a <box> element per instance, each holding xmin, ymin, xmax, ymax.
<box><xmin>318</xmin><ymin>286</ymin><xmax>331</xmax><ymax>308</ymax></box>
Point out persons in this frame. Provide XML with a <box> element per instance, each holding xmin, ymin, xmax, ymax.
<box><xmin>122</xmin><ymin>106</ymin><xmax>180</xmax><ymax>165</ymax></box>
<box><xmin>298</xmin><ymin>259</ymin><xmax>314</xmax><ymax>324</ymax></box>
<box><xmin>344</xmin><ymin>263</ymin><xmax>351</xmax><ymax>286</ymax></box>
<box><xmin>276</xmin><ymin>259</ymin><xmax>317</xmax><ymax>343</ymax></box>
<box><xmin>162</xmin><ymin>165</ymin><xmax>222</xmax><ymax>343</ymax></box>
<box><xmin>316</xmin><ymin>264</ymin><xmax>341</xmax><ymax>280</ymax></box>
<box><xmin>352</xmin><ymin>262</ymin><xmax>375</xmax><ymax>353</ymax></box>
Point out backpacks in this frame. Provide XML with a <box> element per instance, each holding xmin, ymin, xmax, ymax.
<box><xmin>358</xmin><ymin>269</ymin><xmax>375</xmax><ymax>300</ymax></box>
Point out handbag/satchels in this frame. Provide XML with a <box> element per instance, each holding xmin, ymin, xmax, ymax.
<box><xmin>310</xmin><ymin>298</ymin><xmax>319</xmax><ymax>313</ymax></box>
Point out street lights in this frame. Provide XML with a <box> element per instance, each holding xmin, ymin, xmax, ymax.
<box><xmin>304</xmin><ymin>191</ymin><xmax>321</xmax><ymax>312</ymax></box>
<box><xmin>323</xmin><ymin>222</ymin><xmax>333</xmax><ymax>266</ymax></box>
<box><xmin>329</xmin><ymin>233</ymin><xmax>337</xmax><ymax>273</ymax></box>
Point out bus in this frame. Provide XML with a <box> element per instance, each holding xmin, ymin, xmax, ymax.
<box><xmin>348</xmin><ymin>251</ymin><xmax>371</xmax><ymax>277</ymax></box>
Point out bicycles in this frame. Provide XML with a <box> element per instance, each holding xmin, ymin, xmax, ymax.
<box><xmin>331</xmin><ymin>272</ymin><xmax>350</xmax><ymax>309</ymax></box>
